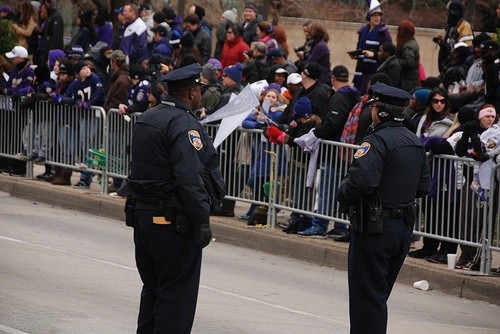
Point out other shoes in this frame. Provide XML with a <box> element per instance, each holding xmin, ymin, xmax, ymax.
<box><xmin>0</xmin><ymin>167</ymin><xmax>499</xmax><ymax>278</ymax></box>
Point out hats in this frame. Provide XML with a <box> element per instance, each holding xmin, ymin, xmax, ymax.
<box><xmin>0</xmin><ymin>0</ymin><xmax>500</xmax><ymax>145</ymax></box>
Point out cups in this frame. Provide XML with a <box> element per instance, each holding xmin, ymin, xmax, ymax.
<box><xmin>447</xmin><ymin>254</ymin><xmax>457</xmax><ymax>269</ymax></box>
<box><xmin>413</xmin><ymin>280</ymin><xmax>429</xmax><ymax>291</ymax></box>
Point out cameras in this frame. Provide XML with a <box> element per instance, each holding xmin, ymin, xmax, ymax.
<box><xmin>246</xmin><ymin>50</ymin><xmax>253</xmax><ymax>57</ymax></box>
<box><xmin>433</xmin><ymin>34</ymin><xmax>442</xmax><ymax>44</ymax></box>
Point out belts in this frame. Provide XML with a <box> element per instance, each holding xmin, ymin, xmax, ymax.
<box><xmin>382</xmin><ymin>208</ymin><xmax>390</xmax><ymax>217</ymax></box>
<box><xmin>135</xmin><ymin>201</ymin><xmax>165</xmax><ymax>211</ymax></box>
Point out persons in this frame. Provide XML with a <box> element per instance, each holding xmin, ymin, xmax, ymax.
<box><xmin>298</xmin><ymin>0</ymin><xmax>500</xmax><ymax>276</ymax></box>
<box><xmin>0</xmin><ymin>0</ymin><xmax>332</xmax><ymax>234</ymax></box>
<box><xmin>334</xmin><ymin>82</ymin><xmax>432</xmax><ymax>334</ymax></box>
<box><xmin>123</xmin><ymin>63</ymin><xmax>226</xmax><ymax>334</ymax></box>
